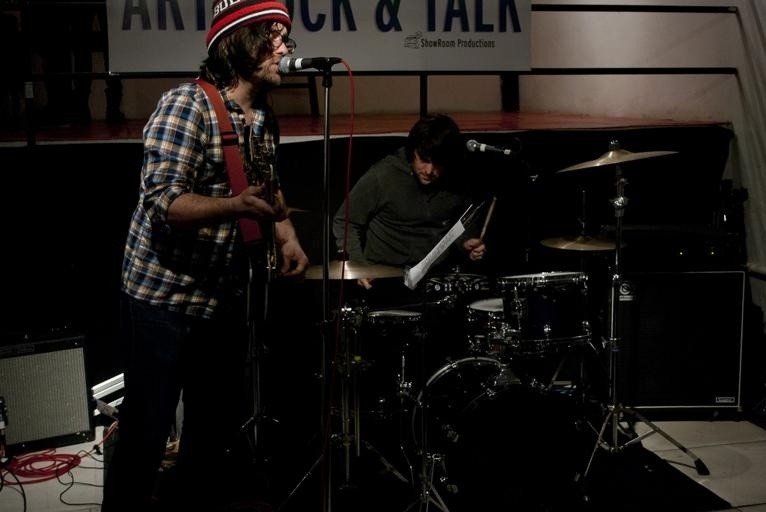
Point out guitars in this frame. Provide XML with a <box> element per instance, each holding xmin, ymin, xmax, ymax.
<box><xmin>241</xmin><ymin>100</ymin><xmax>280</xmax><ymax>280</ymax></box>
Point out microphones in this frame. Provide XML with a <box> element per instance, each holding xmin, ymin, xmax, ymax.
<box><xmin>278</xmin><ymin>56</ymin><xmax>341</xmax><ymax>74</ymax></box>
<box><xmin>466</xmin><ymin>137</ymin><xmax>513</xmax><ymax>158</ymax></box>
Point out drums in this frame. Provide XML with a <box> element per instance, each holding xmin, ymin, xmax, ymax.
<box><xmin>499</xmin><ymin>272</ymin><xmax>592</xmax><ymax>357</ymax></box>
<box><xmin>402</xmin><ymin>356</ymin><xmax>501</xmax><ymax>459</ymax></box>
<box><xmin>366</xmin><ymin>311</ymin><xmax>423</xmax><ymax>349</ymax></box>
<box><xmin>467</xmin><ymin>298</ymin><xmax>504</xmax><ymax>357</ymax></box>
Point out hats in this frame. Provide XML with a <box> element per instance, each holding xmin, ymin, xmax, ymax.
<box><xmin>206</xmin><ymin>0</ymin><xmax>292</xmax><ymax>57</ymax></box>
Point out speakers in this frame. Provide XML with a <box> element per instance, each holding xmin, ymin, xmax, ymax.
<box><xmin>0</xmin><ymin>333</ymin><xmax>95</xmax><ymax>457</ymax></box>
<box><xmin>604</xmin><ymin>268</ymin><xmax>747</xmax><ymax>420</ymax></box>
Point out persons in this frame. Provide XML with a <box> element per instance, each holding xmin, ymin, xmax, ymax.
<box><xmin>332</xmin><ymin>113</ymin><xmax>485</xmax><ymax>290</ymax></box>
<box><xmin>105</xmin><ymin>0</ymin><xmax>312</xmax><ymax>512</ymax></box>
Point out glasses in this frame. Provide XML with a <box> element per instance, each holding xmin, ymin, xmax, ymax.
<box><xmin>268</xmin><ymin>29</ymin><xmax>297</xmax><ymax>54</ymax></box>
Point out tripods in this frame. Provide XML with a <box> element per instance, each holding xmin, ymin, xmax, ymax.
<box><xmin>390</xmin><ymin>271</ymin><xmax>462</xmax><ymax>512</ymax></box>
<box><xmin>240</xmin><ymin>340</ymin><xmax>284</xmax><ymax>466</ymax></box>
<box><xmin>570</xmin><ymin>176</ymin><xmax>710</xmax><ymax>489</ymax></box>
<box><xmin>276</xmin><ymin>300</ymin><xmax>411</xmax><ymax>512</ymax></box>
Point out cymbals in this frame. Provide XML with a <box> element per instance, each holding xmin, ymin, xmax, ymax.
<box><xmin>298</xmin><ymin>250</ymin><xmax>408</xmax><ymax>282</ymax></box>
<box><xmin>540</xmin><ymin>235</ymin><xmax>629</xmax><ymax>250</ymax></box>
<box><xmin>556</xmin><ymin>149</ymin><xmax>678</xmax><ymax>173</ymax></box>
